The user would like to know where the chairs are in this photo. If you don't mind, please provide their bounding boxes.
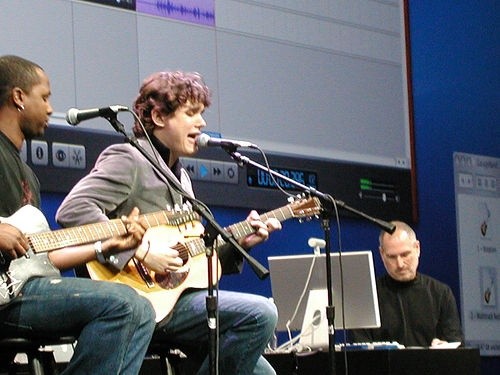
[0,261,199,375]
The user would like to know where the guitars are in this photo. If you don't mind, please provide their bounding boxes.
[0,204,203,305]
[73,191,324,325]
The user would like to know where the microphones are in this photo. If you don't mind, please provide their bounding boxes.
[196,133,258,148]
[66,105,128,126]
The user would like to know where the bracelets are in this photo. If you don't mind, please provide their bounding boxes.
[138,239,150,262]
[95,240,106,264]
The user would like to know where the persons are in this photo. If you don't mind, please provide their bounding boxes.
[0,56,157,375]
[348,221,464,349]
[55,72,281,375]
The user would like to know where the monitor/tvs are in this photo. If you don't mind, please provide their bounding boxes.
[268,251,381,353]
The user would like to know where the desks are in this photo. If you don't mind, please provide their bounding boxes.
[262,348,482,375]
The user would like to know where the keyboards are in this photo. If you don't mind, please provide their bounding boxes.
[334,340,405,351]
